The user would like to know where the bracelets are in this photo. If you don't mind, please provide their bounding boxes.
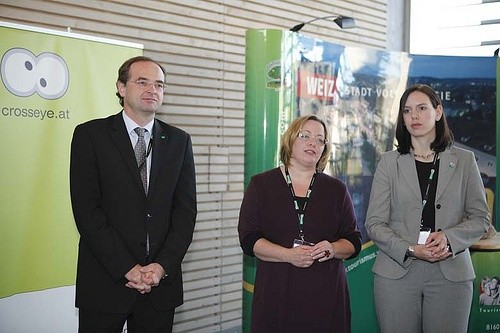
[162,274,168,281]
[407,244,414,259]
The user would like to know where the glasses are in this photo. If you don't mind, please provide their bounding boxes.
[296,131,329,147]
[125,79,168,92]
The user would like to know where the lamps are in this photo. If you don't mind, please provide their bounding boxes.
[289,15,355,32]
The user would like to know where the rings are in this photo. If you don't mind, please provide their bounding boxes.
[323,250,330,258]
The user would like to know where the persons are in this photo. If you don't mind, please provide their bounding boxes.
[69,56,198,333]
[478,277,500,305]
[364,84,491,333]
[237,115,362,333]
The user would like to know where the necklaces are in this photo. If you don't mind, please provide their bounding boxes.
[413,152,434,159]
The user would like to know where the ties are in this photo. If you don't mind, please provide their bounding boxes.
[132,128,148,197]
